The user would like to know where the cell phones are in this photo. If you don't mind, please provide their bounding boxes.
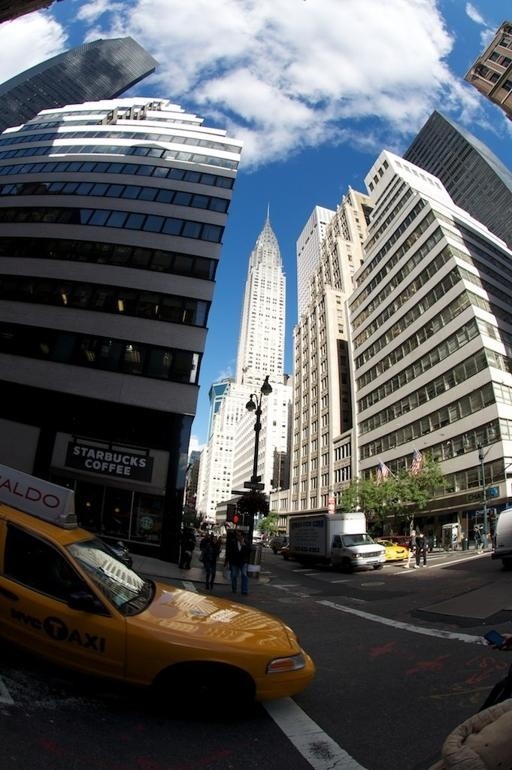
[484,630,504,651]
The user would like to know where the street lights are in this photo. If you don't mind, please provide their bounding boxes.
[245,374,273,492]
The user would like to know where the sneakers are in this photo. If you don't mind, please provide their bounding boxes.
[403,564,427,568]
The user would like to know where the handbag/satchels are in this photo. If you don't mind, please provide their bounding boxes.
[199,552,203,561]
[223,566,232,581]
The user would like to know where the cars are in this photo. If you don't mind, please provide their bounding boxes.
[252,530,290,554]
[373,534,434,562]
[0,462,316,701]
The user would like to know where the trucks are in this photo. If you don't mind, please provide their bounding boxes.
[289,512,385,571]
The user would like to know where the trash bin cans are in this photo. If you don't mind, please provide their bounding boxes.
[247,544,262,579]
[462,538,469,550]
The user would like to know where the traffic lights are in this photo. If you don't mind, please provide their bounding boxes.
[227,503,252,526]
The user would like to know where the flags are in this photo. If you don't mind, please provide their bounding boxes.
[380,461,388,482]
[376,468,381,486]
[410,450,422,475]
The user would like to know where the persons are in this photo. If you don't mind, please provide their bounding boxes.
[452,525,459,551]
[479,633,512,711]
[179,526,196,568]
[403,530,427,569]
[260,532,266,547]
[473,527,480,549]
[227,530,252,595]
[198,532,222,590]
